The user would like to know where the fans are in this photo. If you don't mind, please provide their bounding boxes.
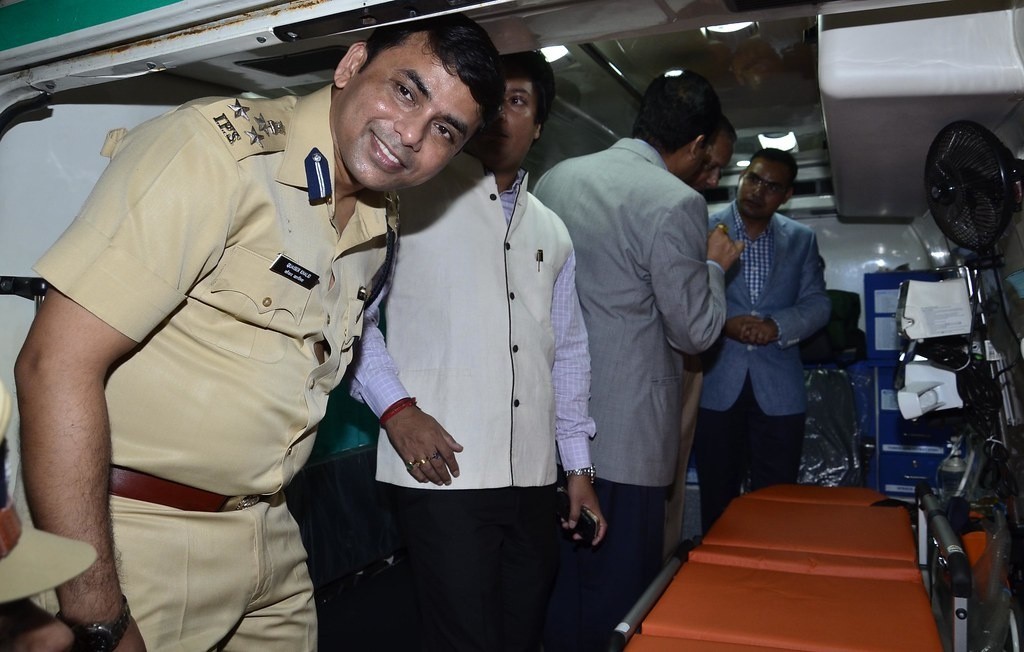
[926,117,1024,256]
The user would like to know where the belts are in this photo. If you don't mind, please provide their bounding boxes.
[108,465,260,513]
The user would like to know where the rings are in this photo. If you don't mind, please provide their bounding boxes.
[716,223,729,234]
[417,458,429,468]
[405,460,418,471]
[428,450,441,461]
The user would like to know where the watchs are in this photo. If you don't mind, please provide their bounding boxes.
[53,594,131,652]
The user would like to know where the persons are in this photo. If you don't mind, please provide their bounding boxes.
[350,50,607,652]
[1,382,99,652]
[15,3,507,652]
[692,147,834,536]
[691,114,738,193]
[533,70,749,652]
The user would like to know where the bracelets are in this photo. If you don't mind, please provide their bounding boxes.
[566,462,596,484]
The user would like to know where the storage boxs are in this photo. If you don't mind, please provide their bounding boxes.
[856,267,961,502]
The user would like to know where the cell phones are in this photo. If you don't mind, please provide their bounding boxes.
[554,483,599,544]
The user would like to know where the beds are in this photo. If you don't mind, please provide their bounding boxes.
[612,481,979,652]
